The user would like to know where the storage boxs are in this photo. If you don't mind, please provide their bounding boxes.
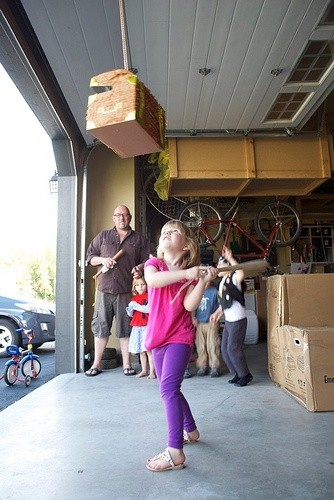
[254,273,334,413]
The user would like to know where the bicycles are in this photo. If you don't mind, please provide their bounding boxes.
[0,329,41,387]
[177,200,302,276]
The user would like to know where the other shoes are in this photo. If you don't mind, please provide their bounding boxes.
[210,367,220,377]
[184,368,191,378]
[198,368,208,375]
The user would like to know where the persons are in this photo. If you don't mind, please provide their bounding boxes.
[126,276,156,379]
[210,245,253,386]
[144,220,220,472]
[85,206,144,376]
[191,282,222,377]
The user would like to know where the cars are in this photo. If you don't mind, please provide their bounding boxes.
[0,296,55,358]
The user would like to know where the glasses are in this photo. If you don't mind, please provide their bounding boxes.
[114,213,130,218]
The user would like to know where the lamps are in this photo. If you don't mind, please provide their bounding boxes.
[48,170,58,195]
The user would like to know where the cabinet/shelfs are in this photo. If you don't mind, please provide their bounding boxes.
[241,220,334,274]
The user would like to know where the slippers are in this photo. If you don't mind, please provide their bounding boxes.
[85,369,102,376]
[181,432,199,444]
[146,449,185,472]
[123,368,135,375]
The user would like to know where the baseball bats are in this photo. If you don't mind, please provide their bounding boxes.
[92,248,125,279]
[218,258,268,273]
